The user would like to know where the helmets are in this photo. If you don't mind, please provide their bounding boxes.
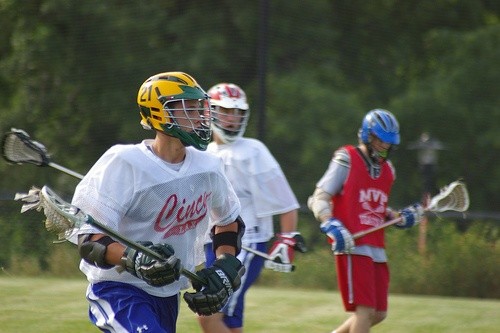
[359,108,402,148]
[135,71,212,151]
[205,82,251,144]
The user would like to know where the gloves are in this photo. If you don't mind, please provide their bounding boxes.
[318,218,357,255]
[183,253,247,316]
[119,240,183,288]
[389,203,424,229]
[263,231,308,274]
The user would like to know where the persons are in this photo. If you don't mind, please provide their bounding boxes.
[308,108,428,333]
[183,82,299,333]
[67,71,241,333]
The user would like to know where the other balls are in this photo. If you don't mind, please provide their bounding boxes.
[45,216,63,234]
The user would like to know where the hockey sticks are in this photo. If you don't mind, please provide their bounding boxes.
[14,186,209,287]
[2,128,277,260]
[328,181,471,244]
